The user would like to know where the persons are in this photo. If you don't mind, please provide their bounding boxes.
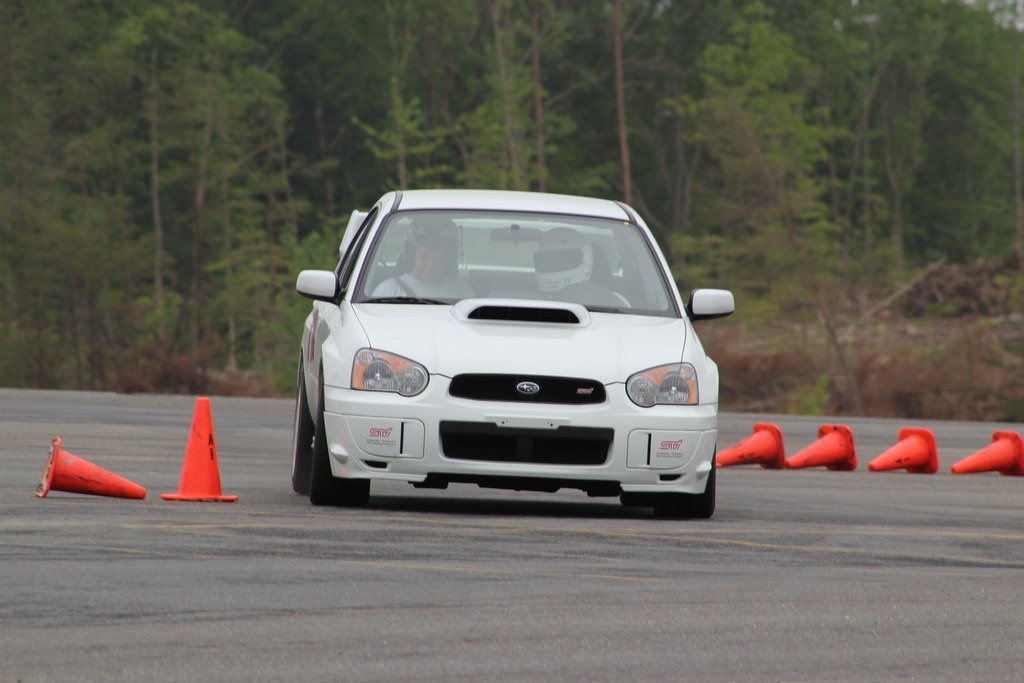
[372,214,476,299]
[523,226,604,305]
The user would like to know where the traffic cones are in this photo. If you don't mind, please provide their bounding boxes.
[35,437,147,499]
[868,427,938,473]
[786,424,857,471]
[161,398,238,500]
[951,430,1024,476]
[715,422,786,469]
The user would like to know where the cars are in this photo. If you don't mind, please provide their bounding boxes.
[292,189,735,519]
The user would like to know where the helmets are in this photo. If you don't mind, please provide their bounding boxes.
[533,227,594,292]
[404,215,461,249]
